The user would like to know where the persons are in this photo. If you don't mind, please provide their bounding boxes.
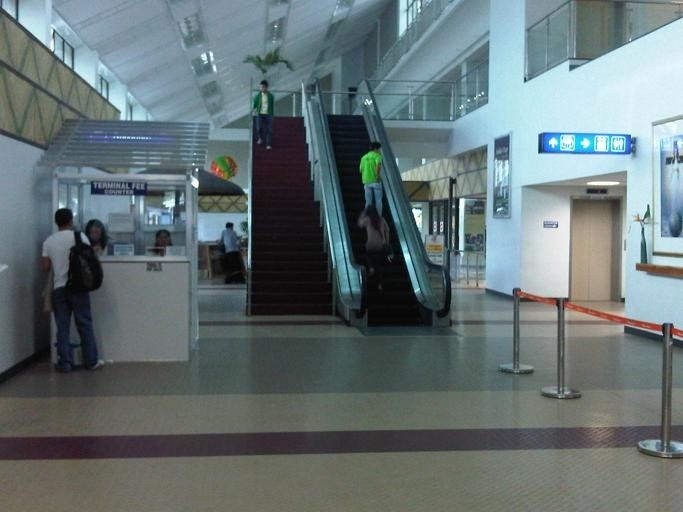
[358,205,390,290]
[251,80,274,151]
[41,209,105,373]
[218,222,241,254]
[153,230,172,256]
[85,219,112,256]
[359,142,383,216]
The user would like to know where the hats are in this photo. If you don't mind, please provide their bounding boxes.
[369,142,381,150]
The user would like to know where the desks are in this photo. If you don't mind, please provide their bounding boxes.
[197,235,247,279]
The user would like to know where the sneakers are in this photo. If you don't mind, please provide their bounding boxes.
[257,138,272,150]
[91,360,104,369]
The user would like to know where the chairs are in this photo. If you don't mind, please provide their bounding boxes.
[220,236,245,284]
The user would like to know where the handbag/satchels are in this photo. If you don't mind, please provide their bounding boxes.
[380,251,395,264]
[66,230,103,294]
[217,243,225,254]
